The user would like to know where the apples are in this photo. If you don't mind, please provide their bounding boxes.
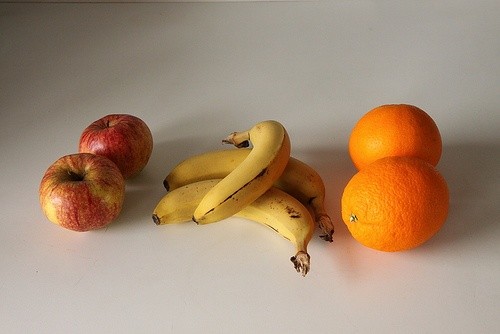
[78,114,153,178]
[38,153,124,232]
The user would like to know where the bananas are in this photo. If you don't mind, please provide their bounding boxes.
[152,179,316,277]
[163,148,334,242]
[193,119,291,225]
